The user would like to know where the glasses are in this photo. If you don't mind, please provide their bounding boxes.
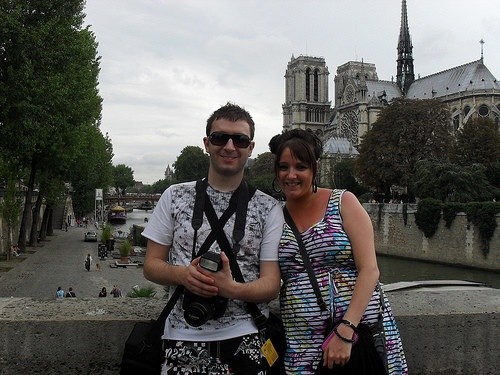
[209,131,252,150]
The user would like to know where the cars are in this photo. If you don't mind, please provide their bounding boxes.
[83,231,98,242]
[105,206,127,225]
[122,202,154,212]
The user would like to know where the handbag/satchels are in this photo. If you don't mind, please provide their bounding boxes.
[120,319,163,375]
[314,318,388,375]
[264,309,287,374]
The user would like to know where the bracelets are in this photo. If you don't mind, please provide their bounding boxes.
[335,329,354,343]
[338,320,359,333]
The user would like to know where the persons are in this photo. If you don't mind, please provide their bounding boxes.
[63,220,68,232]
[144,217,148,223]
[76,214,99,229]
[84,253,93,271]
[55,286,65,298]
[110,284,122,298]
[141,104,287,375]
[99,287,107,298]
[269,127,411,375]
[66,287,76,297]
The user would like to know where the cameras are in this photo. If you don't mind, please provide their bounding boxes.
[182,252,228,327]
